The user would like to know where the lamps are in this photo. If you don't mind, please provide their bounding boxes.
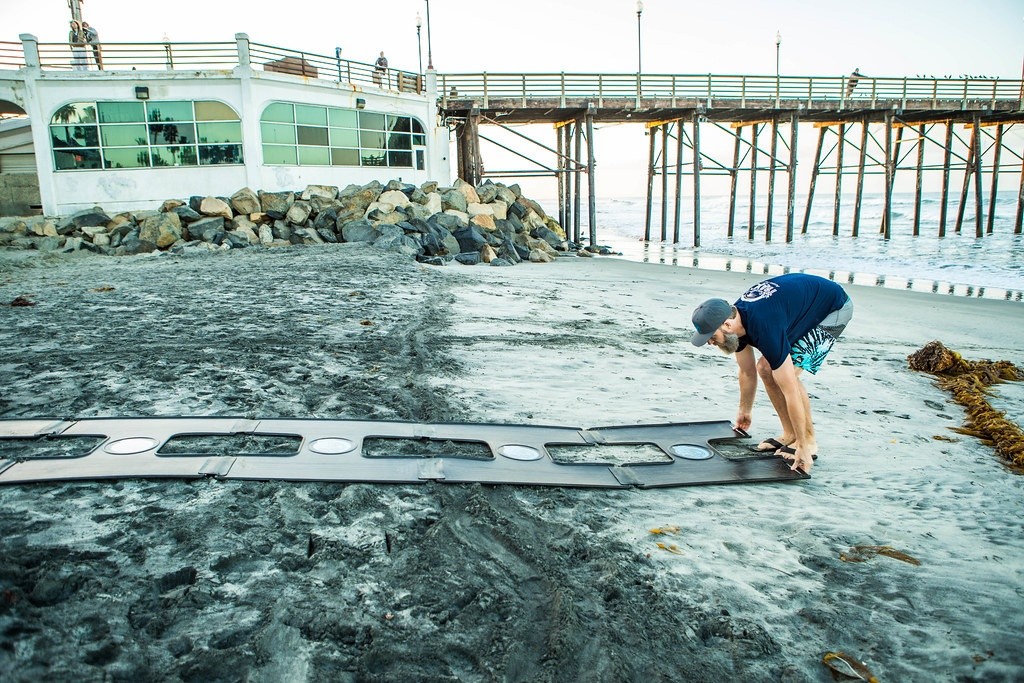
[357,99,366,109]
[135,87,149,99]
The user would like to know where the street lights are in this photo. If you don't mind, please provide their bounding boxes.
[774,29,784,110]
[636,0,645,98]
[414,12,424,91]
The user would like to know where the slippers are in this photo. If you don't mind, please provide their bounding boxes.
[748,438,818,463]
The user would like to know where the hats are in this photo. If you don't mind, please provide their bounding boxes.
[691,298,731,347]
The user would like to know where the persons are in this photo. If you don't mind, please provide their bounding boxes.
[374,51,389,87]
[68,20,105,73]
[689,271,854,476]
[845,68,869,98]
